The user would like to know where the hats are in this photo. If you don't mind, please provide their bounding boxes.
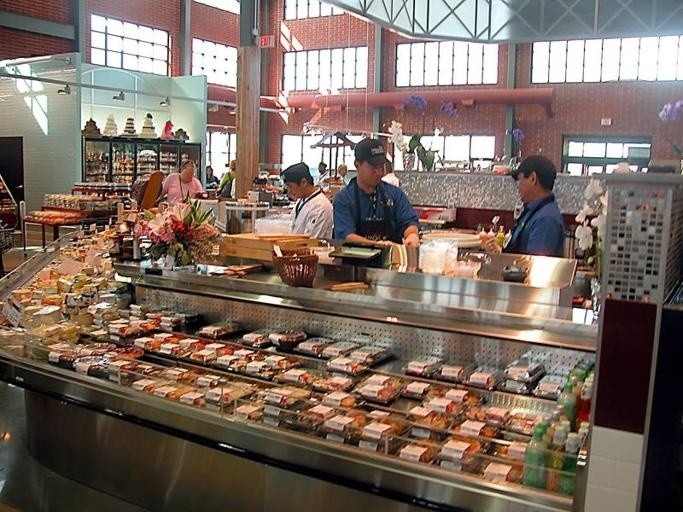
[354,138,389,166]
[281,162,309,181]
[509,155,556,178]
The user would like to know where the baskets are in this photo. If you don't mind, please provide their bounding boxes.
[272,249,318,286]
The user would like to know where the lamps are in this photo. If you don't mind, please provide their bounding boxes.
[58,84,72,95]
[160,97,172,107]
[113,92,125,101]
[208,104,219,111]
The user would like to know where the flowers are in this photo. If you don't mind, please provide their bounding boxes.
[387,120,422,152]
[133,191,224,273]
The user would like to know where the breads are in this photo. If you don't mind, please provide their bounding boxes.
[1,276,561,483]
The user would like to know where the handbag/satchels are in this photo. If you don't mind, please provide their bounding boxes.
[221,172,232,197]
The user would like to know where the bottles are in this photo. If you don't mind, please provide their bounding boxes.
[476,222,512,251]
[523,358,596,495]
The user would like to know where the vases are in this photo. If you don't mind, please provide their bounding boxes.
[403,152,415,172]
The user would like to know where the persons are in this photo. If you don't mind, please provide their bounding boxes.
[217,161,237,197]
[157,162,203,213]
[283,162,334,240]
[205,166,218,187]
[316,163,329,183]
[495,154,566,258]
[336,164,349,188]
[382,160,399,188]
[332,138,420,250]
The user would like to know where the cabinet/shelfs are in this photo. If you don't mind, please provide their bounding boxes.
[22,187,120,250]
[80,135,201,192]
[1,230,600,511]
